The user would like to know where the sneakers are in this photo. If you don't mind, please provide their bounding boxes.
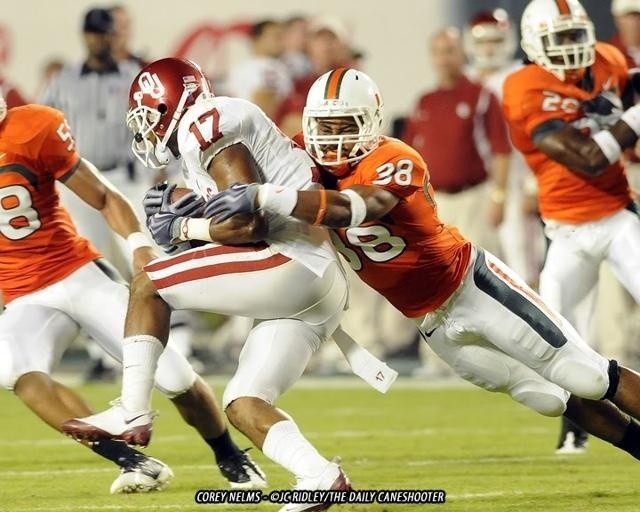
[216,454,267,495]
[57,413,156,447]
[555,418,591,460]
[108,458,172,494]
[276,463,355,508]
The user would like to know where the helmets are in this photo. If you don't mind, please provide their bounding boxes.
[462,10,516,72]
[302,68,383,161]
[124,56,215,172]
[517,0,597,79]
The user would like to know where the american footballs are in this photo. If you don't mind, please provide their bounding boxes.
[170,188,211,246]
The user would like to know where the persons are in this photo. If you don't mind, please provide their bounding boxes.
[2,0,640,511]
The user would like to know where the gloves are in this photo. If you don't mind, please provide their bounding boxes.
[145,182,254,248]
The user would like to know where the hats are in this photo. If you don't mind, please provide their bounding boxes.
[84,8,115,35]
